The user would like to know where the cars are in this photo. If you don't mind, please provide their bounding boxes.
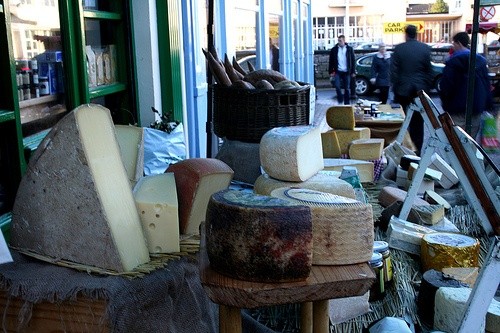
[329,50,447,97]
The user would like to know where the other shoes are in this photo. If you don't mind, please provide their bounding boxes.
[338,94,343,104]
[344,97,351,105]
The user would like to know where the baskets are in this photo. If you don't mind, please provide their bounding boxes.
[210,80,309,143]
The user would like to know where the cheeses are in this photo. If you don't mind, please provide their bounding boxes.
[10,102,233,275]
[205,106,480,333]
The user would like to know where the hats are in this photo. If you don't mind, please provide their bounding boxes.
[379,42,386,47]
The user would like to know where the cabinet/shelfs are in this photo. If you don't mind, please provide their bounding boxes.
[0,0,135,244]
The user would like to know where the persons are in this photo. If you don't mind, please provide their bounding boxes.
[439,32,490,142]
[327,34,356,105]
[388,25,434,153]
[371,42,394,104]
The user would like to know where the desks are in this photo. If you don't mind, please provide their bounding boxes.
[354,104,405,138]
[199,222,376,333]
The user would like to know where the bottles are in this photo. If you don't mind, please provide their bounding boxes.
[373,240,393,288]
[367,252,384,303]
[14,58,49,102]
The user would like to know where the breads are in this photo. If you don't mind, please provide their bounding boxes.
[202,47,300,90]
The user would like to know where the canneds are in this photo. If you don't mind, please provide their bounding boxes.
[16,58,49,100]
